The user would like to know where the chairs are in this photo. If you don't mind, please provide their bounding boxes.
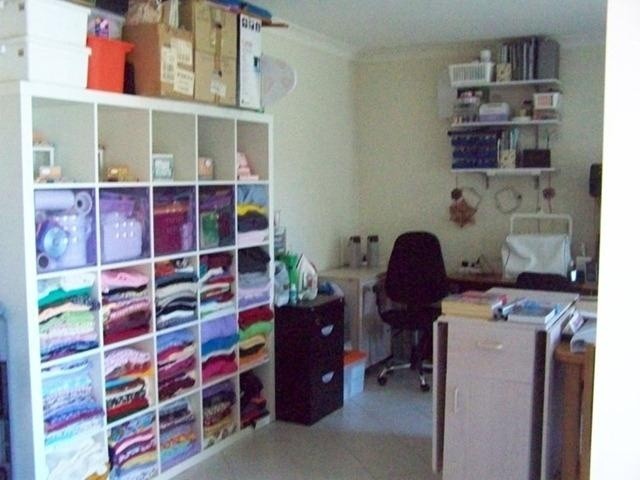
[372,229,449,393]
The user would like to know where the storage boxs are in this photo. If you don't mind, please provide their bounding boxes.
[344,352,367,399]
[0,0,262,115]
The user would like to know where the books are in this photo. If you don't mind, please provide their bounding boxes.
[500,40,559,79]
[508,306,556,324]
[440,291,506,320]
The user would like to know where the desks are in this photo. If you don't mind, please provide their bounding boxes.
[556,336,591,480]
[450,272,505,295]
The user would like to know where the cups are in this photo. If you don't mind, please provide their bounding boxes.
[500,148,516,169]
[496,63,513,82]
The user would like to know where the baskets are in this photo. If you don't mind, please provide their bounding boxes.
[101,217,142,262]
[92,191,136,218]
[52,213,87,269]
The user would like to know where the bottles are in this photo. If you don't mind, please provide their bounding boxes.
[365,234,381,269]
[520,109,528,116]
[567,260,577,288]
[460,261,469,276]
[347,234,362,270]
[290,283,298,304]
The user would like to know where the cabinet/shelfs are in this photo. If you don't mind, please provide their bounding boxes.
[318,260,391,373]
[431,289,578,480]
[276,296,345,427]
[0,81,278,479]
[450,80,563,175]
